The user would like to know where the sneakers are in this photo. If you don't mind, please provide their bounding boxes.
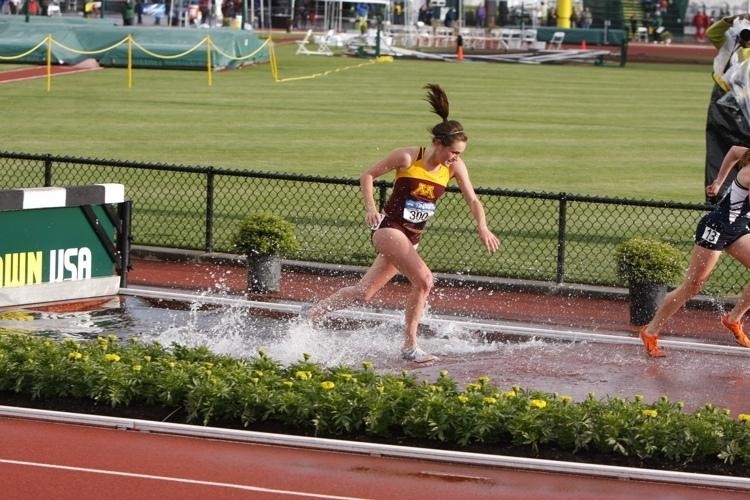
[721,314,750,348]
[300,304,313,321]
[639,325,667,358]
[400,346,437,363]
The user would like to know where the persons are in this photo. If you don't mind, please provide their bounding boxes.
[9,0,234,27]
[353,0,593,34]
[456,35,464,61]
[299,120,500,366]
[630,0,750,42]
[704,13,750,204]
[639,145,750,357]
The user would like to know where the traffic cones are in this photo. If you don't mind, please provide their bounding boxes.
[580,39,587,49]
[457,46,465,62]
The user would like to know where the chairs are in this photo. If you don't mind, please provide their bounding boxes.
[294,25,569,57]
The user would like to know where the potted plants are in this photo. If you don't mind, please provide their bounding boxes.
[613,238,690,330]
[230,210,299,292]
